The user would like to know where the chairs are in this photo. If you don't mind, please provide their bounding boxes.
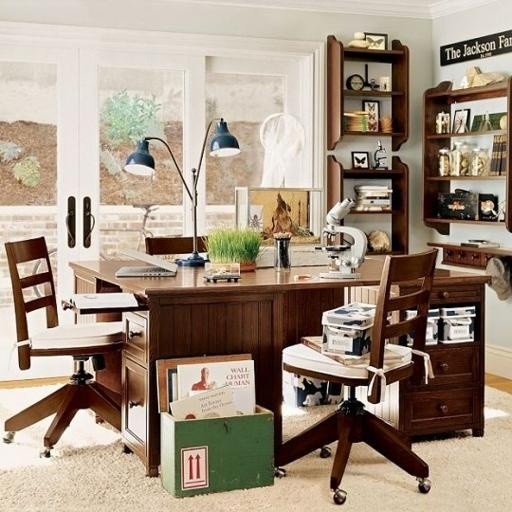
[269,242,438,504]
[143,234,209,262]
[5,235,127,455]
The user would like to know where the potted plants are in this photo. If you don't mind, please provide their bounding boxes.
[202,224,262,274]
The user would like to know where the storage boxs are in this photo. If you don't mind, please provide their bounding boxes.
[319,299,393,357]
[286,367,344,408]
[435,189,498,222]
[443,302,478,344]
[401,308,441,347]
[153,404,276,500]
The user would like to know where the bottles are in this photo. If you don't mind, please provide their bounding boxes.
[436,141,489,177]
[273,231,292,273]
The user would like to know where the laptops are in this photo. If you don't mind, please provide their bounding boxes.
[115,249,178,277]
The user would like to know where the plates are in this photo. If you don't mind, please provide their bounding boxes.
[369,230,389,250]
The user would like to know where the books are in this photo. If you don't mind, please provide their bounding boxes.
[71,291,139,310]
[167,359,257,418]
[300,334,404,369]
[489,134,509,177]
[353,186,392,212]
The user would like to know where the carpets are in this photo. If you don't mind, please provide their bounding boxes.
[1,375,511,510]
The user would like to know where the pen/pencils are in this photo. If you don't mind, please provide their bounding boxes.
[273,231,293,269]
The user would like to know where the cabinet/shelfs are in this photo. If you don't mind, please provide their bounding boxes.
[422,72,512,236]
[326,155,412,256]
[325,30,412,151]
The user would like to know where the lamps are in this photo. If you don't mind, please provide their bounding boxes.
[121,113,240,268]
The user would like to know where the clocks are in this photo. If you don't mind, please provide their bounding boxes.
[346,73,365,91]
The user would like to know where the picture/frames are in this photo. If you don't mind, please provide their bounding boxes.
[352,151,371,170]
[361,99,380,133]
[364,32,389,51]
[453,108,471,134]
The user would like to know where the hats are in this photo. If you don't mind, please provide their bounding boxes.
[486,257,510,300]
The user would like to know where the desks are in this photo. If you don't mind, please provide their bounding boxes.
[66,246,496,441]
